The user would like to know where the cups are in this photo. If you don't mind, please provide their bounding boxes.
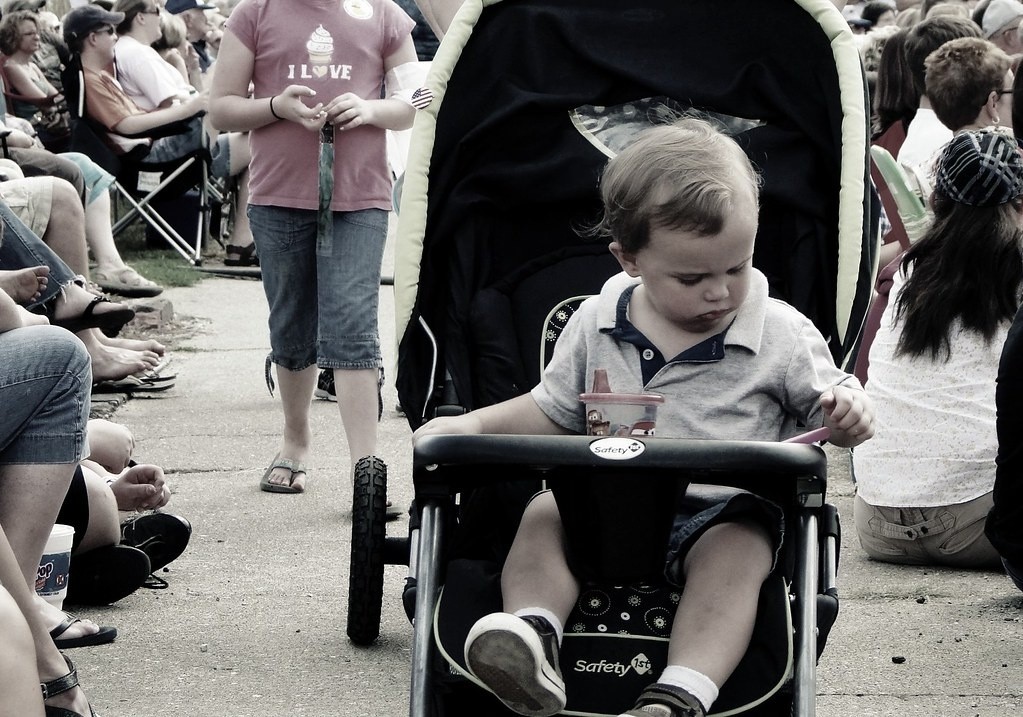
[577,370,664,437]
[36,523,75,611]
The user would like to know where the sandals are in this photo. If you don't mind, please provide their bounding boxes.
[49,610,117,649]
[40,650,98,717]
[94,266,164,298]
[224,241,260,267]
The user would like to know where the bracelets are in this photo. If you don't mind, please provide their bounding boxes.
[270,96,285,121]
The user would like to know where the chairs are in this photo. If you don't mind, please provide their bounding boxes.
[0,54,231,267]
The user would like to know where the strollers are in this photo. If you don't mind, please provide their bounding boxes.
[346,1,898,717]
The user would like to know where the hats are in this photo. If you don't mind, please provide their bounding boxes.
[165,0,215,14]
[981,0,1023,41]
[934,130,1023,208]
[63,4,125,43]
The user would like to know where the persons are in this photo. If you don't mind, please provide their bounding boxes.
[209,0,423,515]
[0,0,260,717]
[833,0,1023,594]
[409,120,877,717]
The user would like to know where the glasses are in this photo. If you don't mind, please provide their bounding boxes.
[94,26,113,35]
[21,29,41,36]
[141,7,159,16]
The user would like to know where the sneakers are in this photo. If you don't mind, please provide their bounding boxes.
[464,612,567,717]
[617,683,706,717]
[315,372,337,401]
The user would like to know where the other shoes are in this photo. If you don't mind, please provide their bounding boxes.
[65,513,191,611]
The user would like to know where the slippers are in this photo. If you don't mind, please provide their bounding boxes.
[51,296,135,334]
[260,451,306,493]
[91,377,175,394]
[140,369,176,383]
